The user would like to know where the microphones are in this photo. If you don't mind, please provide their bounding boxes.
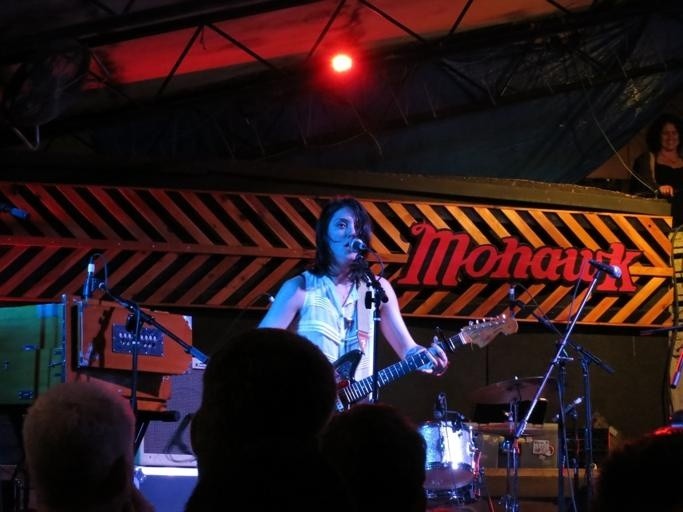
[83,263,97,302]
[348,238,366,256]
[508,288,516,319]
[590,258,621,279]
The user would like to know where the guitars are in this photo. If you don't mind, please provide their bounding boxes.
[335,310,520,422]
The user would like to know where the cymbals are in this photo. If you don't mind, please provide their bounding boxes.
[478,426,545,438]
[470,376,555,404]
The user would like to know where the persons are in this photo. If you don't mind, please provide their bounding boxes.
[312,402,429,511]
[587,425,683,512]
[630,113,683,231]
[256,197,450,416]
[183,326,337,511]
[22,379,158,512]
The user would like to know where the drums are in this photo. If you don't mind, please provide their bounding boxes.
[416,418,476,492]
[426,485,480,503]
